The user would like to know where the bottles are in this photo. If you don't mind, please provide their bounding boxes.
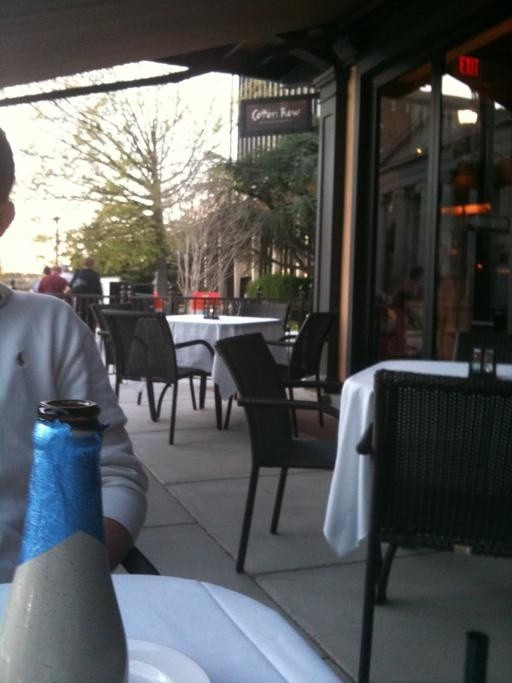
[0,398,130,683]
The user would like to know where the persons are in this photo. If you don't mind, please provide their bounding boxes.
[390,265,424,308]
[32,258,104,338]
[0,126,149,584]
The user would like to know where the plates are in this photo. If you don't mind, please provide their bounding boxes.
[124,639,211,683]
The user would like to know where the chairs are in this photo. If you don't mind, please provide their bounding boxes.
[36,280,159,357]
[224,310,342,429]
[99,308,223,443]
[213,332,334,574]
[194,301,292,411]
[356,369,511,682]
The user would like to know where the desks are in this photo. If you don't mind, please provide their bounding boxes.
[322,358,510,562]
[159,311,285,427]
[0,571,355,683]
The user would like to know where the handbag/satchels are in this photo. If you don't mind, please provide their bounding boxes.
[71,268,87,292]
